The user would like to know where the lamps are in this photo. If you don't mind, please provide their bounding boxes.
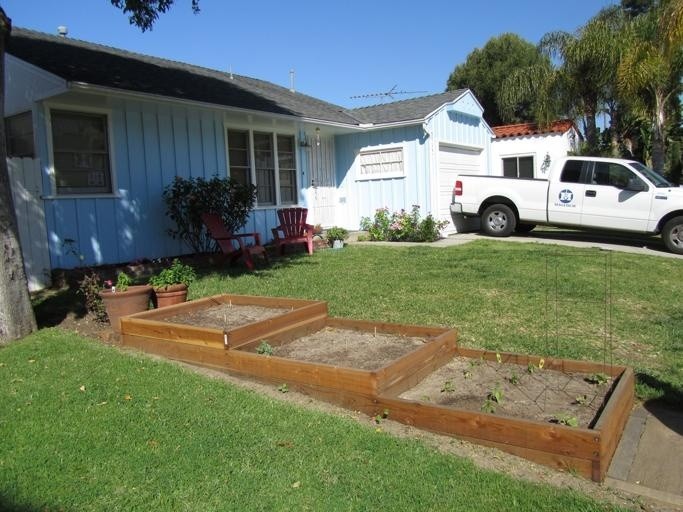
[315,125,320,147]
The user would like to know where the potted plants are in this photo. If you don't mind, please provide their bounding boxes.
[97,257,196,336]
[160,176,258,271]
[326,225,350,249]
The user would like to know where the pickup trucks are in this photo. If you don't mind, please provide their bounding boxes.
[451,155,682,254]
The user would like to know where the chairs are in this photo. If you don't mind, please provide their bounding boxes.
[202,206,314,271]
